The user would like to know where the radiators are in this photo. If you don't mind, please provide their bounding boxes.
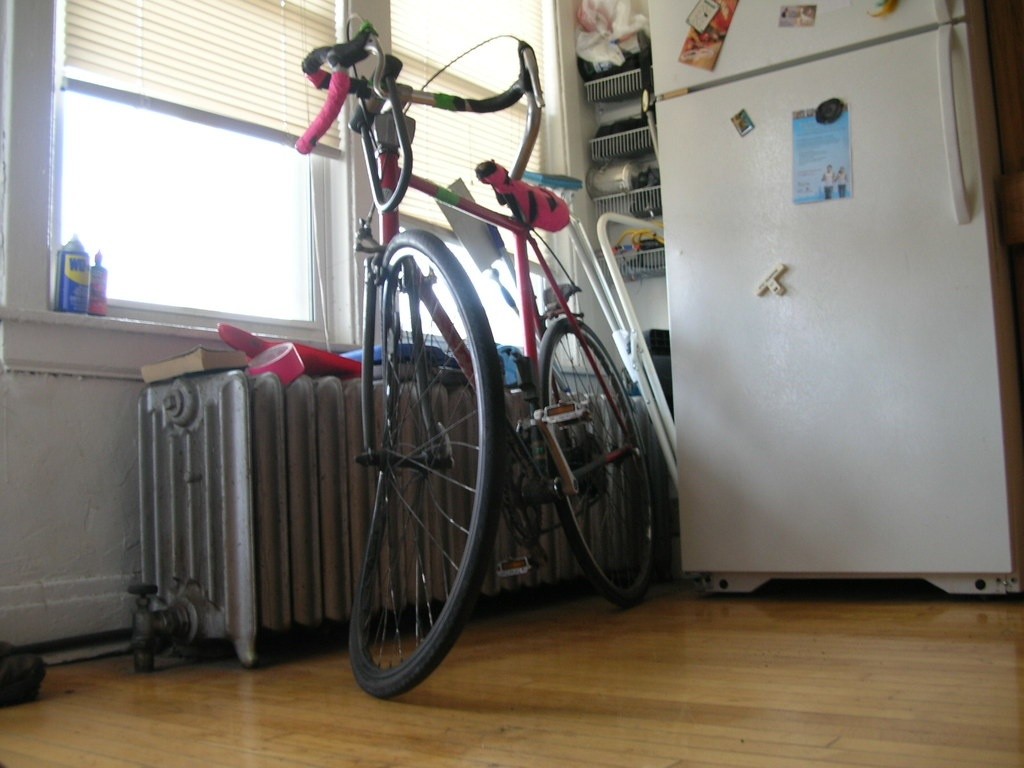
[126,365,674,676]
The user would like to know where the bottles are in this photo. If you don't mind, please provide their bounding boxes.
[54,234,88,315]
[86,251,108,317]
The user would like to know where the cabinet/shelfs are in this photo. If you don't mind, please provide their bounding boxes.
[577,29,665,282]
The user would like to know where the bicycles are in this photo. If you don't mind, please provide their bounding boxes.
[292,13,672,703]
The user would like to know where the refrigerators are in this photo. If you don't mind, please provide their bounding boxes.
[650,1,1023,599]
